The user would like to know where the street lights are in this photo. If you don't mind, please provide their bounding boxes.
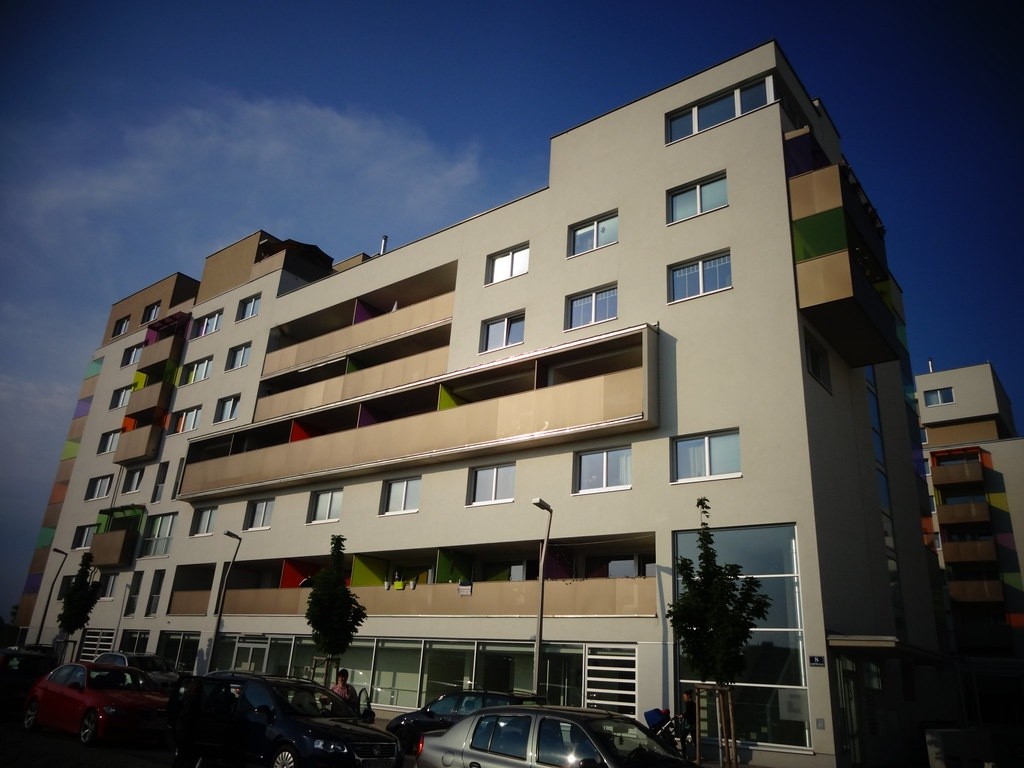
[531,497,552,698]
[204,529,242,677]
[32,546,68,642]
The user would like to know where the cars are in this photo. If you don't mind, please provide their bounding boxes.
[414,704,705,768]
[0,647,52,722]
[381,692,564,756]
[24,661,171,747]
[169,670,403,768]
[90,652,181,680]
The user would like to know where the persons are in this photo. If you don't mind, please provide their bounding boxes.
[319,669,357,717]
[676,691,696,760]
